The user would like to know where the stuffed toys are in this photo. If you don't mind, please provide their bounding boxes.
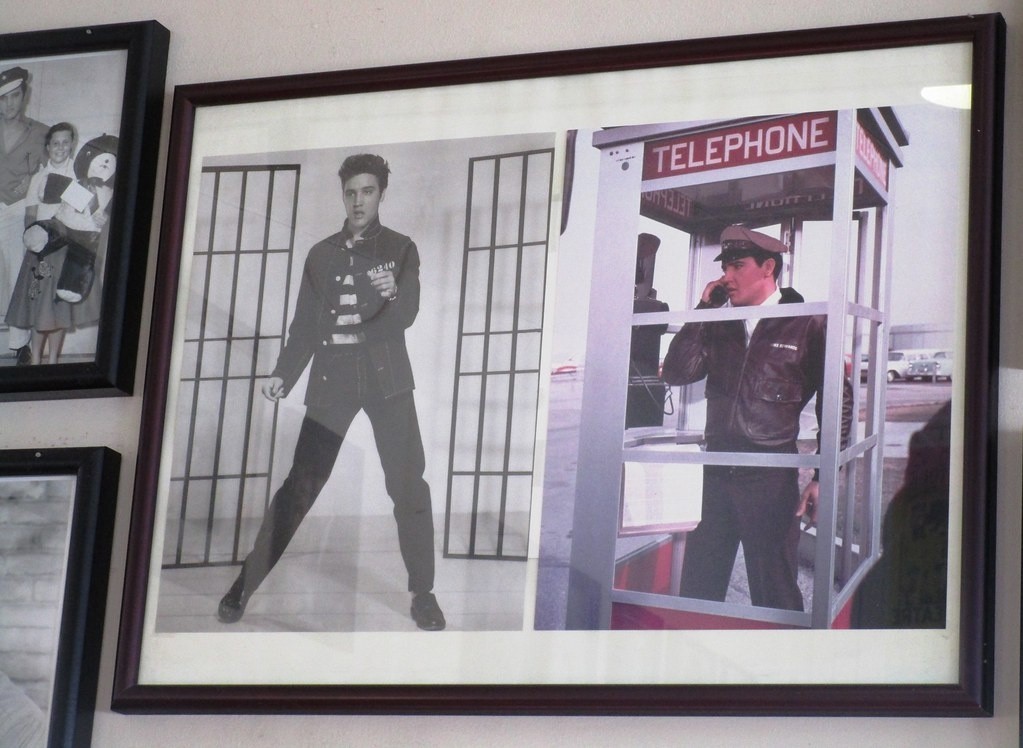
[23,135,119,303]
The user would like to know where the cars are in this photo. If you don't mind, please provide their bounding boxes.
[844,346,955,381]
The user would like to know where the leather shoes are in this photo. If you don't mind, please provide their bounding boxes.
[218,578,252,624]
[411,592,446,632]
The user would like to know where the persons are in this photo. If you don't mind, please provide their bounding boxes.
[662,226,853,614]
[218,155,447,631]
[0,66,97,367]
[851,399,951,631]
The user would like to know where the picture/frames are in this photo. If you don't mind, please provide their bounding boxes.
[0,446,122,748]
[0,20,172,403]
[111,11,1008,717]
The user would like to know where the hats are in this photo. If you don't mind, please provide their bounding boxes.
[712,226,788,262]
[0,67,28,98]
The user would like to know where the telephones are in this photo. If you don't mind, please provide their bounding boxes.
[709,284,730,307]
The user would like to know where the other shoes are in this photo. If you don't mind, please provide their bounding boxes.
[13,345,31,367]
